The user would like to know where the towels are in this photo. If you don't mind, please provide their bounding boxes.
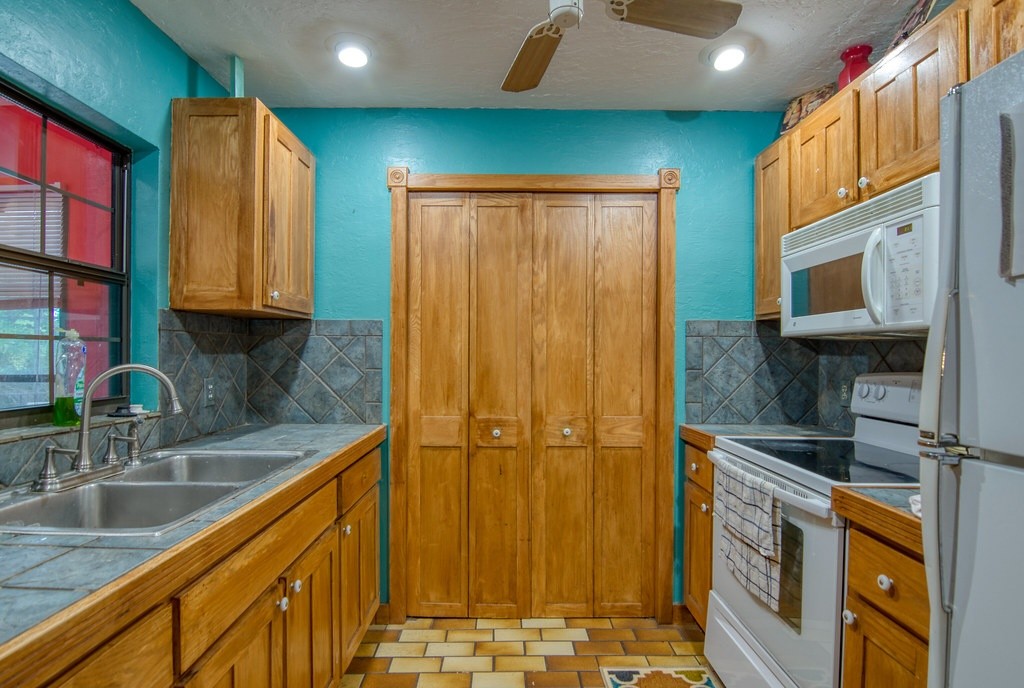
[712,456,782,614]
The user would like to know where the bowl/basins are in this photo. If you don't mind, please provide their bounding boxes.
[908,495,921,519]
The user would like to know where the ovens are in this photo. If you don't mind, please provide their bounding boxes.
[704,446,846,688]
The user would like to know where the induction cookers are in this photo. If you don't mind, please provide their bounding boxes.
[714,372,923,499]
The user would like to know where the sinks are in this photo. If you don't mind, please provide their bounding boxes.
[0,483,244,529]
[101,454,298,482]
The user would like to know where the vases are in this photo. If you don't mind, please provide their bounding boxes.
[839,44,872,92]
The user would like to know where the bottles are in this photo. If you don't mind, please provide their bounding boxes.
[53,326,86,428]
[838,44,872,92]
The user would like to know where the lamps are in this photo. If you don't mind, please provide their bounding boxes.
[336,41,370,67]
[710,45,745,72]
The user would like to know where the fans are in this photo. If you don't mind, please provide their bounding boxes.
[501,0,743,93]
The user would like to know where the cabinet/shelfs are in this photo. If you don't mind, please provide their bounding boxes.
[754,137,790,320]
[337,447,382,676]
[968,0,1024,79]
[843,527,930,688]
[170,97,315,319]
[45,602,174,688]
[172,477,341,688]
[684,445,714,633]
[790,9,967,229]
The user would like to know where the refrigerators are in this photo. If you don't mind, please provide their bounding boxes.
[917,47,1024,688]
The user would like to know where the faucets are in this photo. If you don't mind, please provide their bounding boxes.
[70,364,185,470]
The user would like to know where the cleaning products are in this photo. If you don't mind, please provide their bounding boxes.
[52,326,87,427]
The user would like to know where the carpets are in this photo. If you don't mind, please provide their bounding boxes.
[599,665,719,688]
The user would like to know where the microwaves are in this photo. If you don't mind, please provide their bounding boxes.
[780,172,940,341]
